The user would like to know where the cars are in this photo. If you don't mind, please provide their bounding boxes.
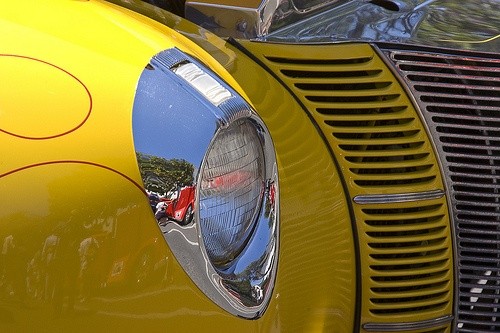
[165,186,196,226]
[0,0,500,333]
